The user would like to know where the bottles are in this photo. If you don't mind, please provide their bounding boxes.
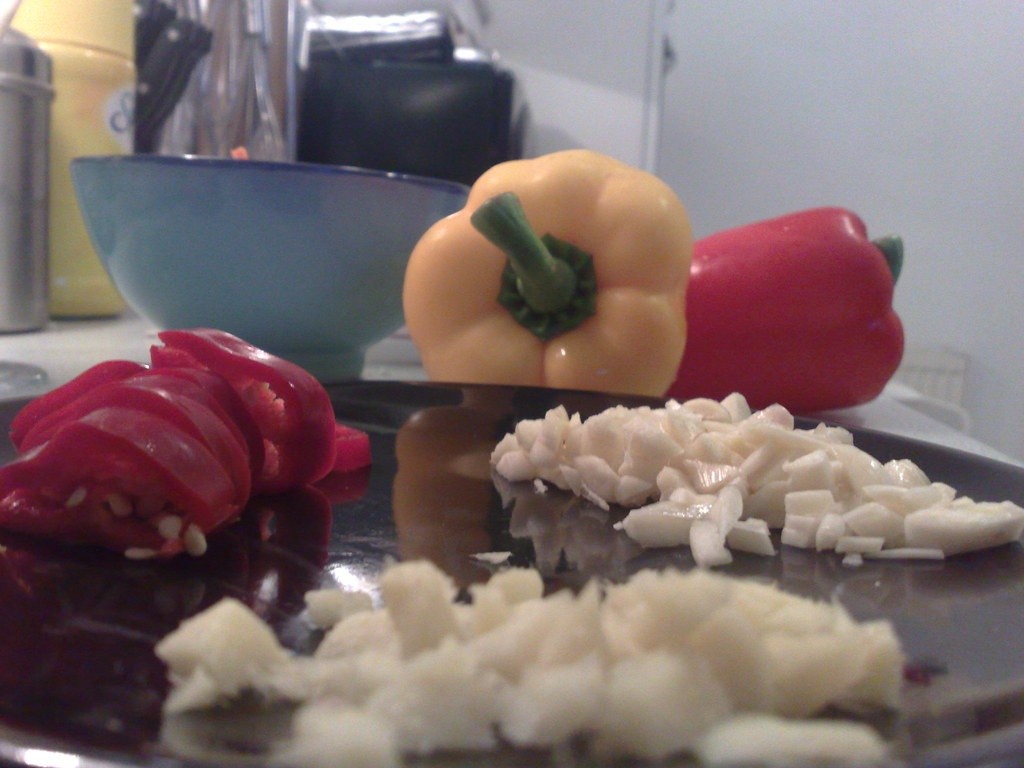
[8,0,148,317]
[0,25,55,334]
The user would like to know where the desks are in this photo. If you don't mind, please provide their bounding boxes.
[0,315,1024,768]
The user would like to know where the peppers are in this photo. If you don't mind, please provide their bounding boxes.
[0,328,370,557]
[402,150,693,396]
[668,208,906,412]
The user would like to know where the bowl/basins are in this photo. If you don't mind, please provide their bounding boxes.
[69,154,471,382]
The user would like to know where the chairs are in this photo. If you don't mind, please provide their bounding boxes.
[893,342,970,406]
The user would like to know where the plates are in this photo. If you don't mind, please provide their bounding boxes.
[0,380,1024,768]
